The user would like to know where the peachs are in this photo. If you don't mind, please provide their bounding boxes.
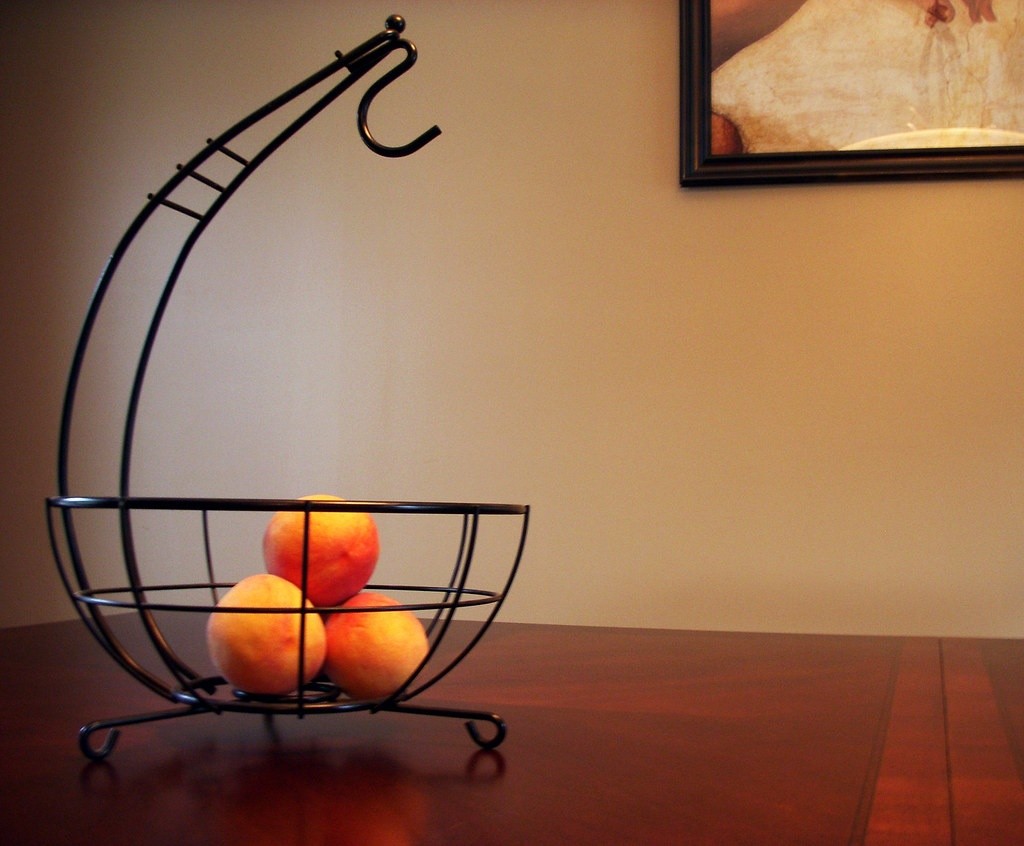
[206,494,436,703]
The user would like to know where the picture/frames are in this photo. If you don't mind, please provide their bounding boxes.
[678,0,1024,187]
